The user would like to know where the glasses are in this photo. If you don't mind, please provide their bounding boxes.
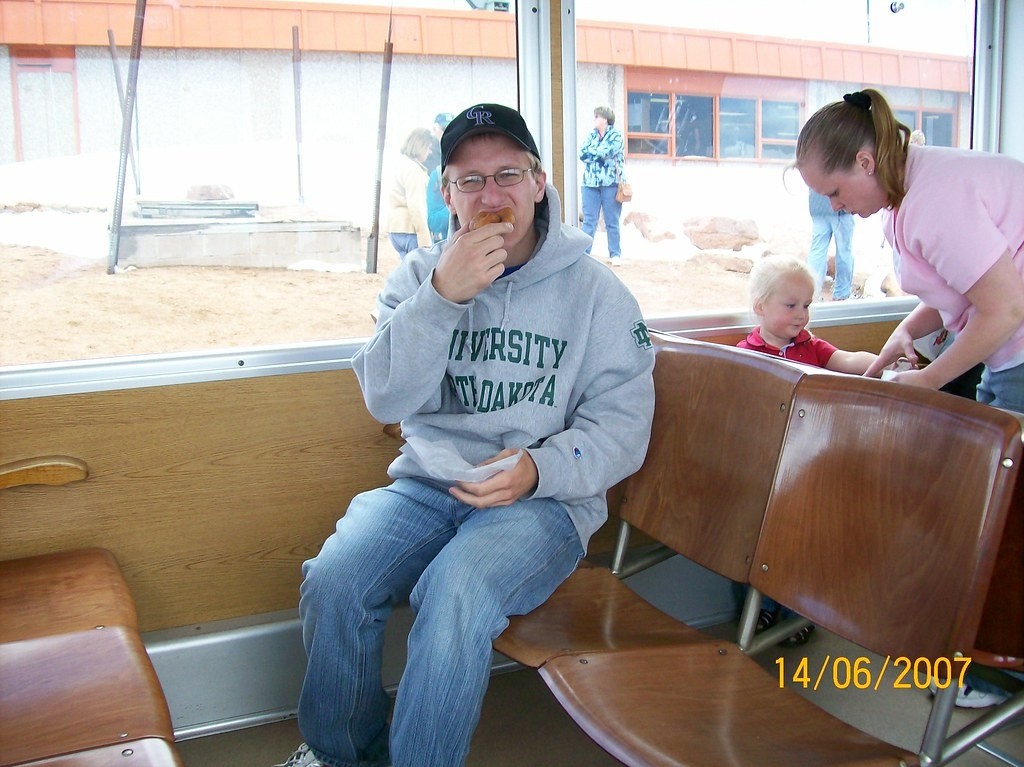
[448,168,532,193]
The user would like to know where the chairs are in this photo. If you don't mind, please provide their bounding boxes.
[0,452,186,767]
[492,330,1023,767]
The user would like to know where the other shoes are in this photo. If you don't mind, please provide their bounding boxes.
[926,676,1008,708]
[277,743,321,767]
[610,256,621,266]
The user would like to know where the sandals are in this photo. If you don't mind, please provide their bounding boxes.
[779,625,814,647]
[735,605,774,633]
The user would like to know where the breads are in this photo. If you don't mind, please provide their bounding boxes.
[468,208,515,231]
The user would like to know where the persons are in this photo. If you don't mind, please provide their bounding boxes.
[796,88,1024,708]
[274,103,654,767]
[386,128,432,261]
[427,166,450,244]
[909,129,925,146]
[578,106,625,265]
[807,187,855,301]
[736,255,919,646]
[422,113,455,177]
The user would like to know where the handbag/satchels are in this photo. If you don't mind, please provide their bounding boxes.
[615,183,633,202]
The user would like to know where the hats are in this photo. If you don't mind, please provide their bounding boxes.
[441,103,541,174]
[435,111,457,131]
